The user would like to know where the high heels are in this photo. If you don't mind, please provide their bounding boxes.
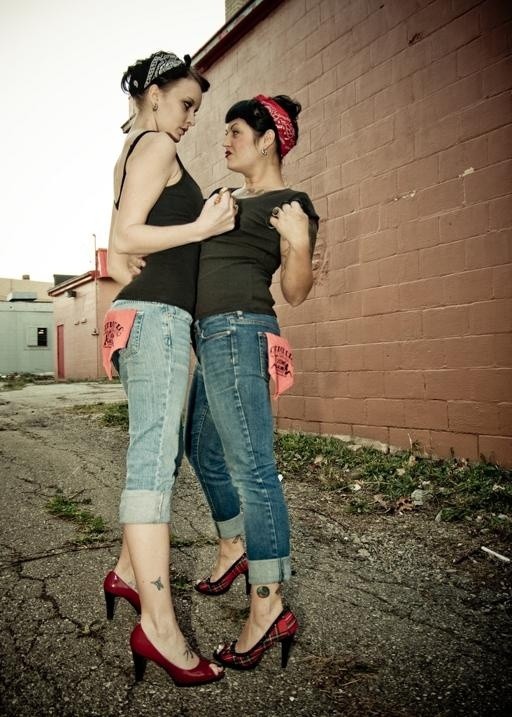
[213,607,297,670]
[104,571,141,620]
[130,624,225,687]
[194,552,251,596]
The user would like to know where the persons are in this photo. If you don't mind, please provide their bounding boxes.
[100,42,240,689]
[124,92,320,673]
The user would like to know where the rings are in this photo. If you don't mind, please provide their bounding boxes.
[272,206,280,216]
